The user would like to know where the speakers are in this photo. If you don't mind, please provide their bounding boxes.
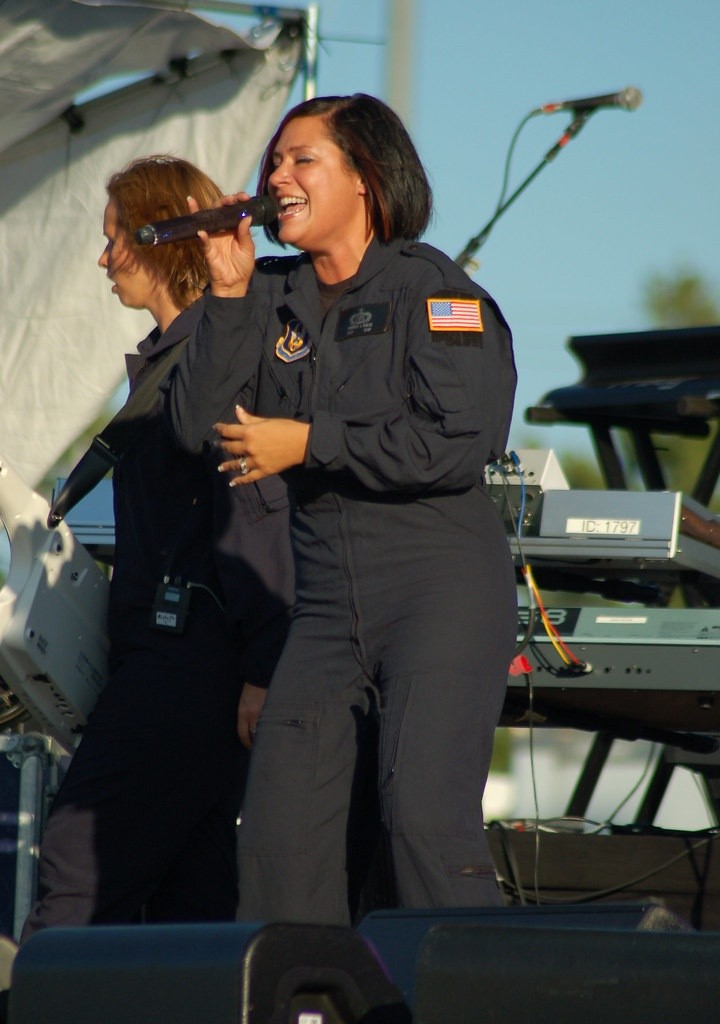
[2,900,719,1024]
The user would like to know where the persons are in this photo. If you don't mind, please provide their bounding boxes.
[186,93,522,924]
[13,154,295,988]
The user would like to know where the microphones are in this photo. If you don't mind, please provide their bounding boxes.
[533,85,643,120]
[132,195,279,246]
